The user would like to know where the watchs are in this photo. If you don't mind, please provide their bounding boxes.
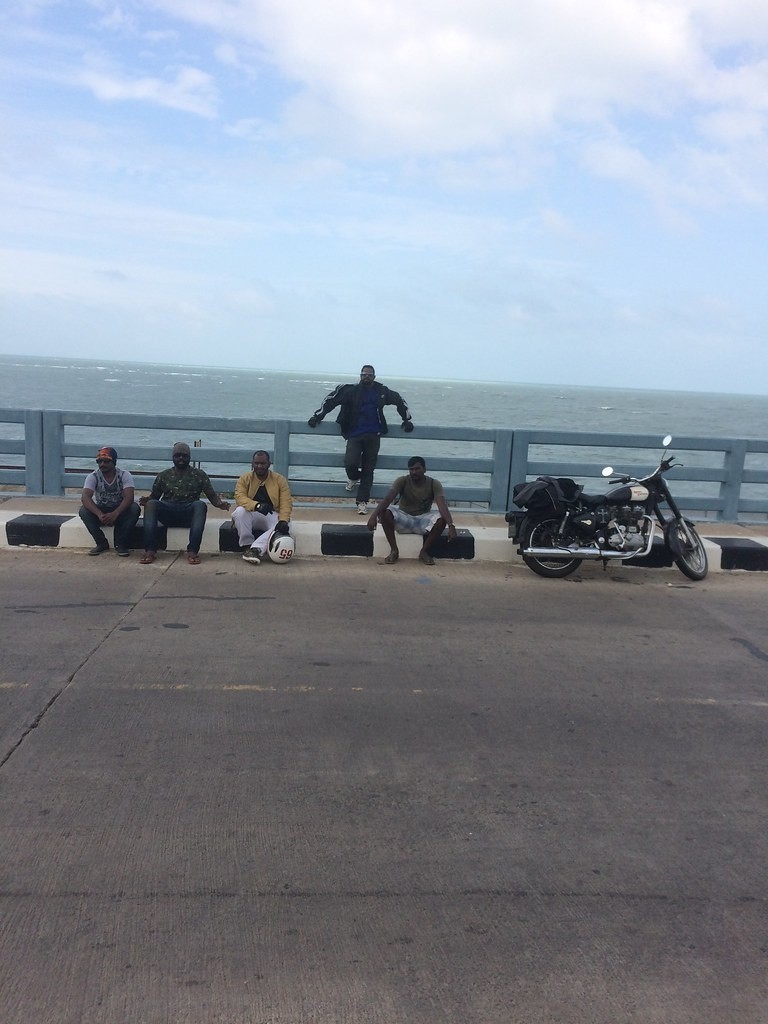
[449,523,455,527]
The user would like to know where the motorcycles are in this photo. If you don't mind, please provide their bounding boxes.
[519,435,710,579]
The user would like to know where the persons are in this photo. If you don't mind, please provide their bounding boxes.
[308,365,414,514]
[79,447,141,557]
[139,442,231,564]
[231,450,292,563]
[366,455,457,565]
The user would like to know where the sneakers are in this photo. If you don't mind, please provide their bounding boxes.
[358,502,367,515]
[345,478,359,491]
[242,548,260,565]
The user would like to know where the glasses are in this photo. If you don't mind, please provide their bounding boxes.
[97,459,111,463]
[360,373,373,377]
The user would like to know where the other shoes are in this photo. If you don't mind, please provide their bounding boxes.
[140,550,157,563]
[419,552,434,565]
[187,552,200,564]
[88,542,109,555]
[115,545,129,556]
[385,551,399,563]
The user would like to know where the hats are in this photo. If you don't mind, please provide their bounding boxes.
[172,442,190,458]
[96,447,117,465]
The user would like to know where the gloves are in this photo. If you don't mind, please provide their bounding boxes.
[308,416,321,428]
[274,521,289,534]
[401,421,413,432]
[255,503,275,516]
